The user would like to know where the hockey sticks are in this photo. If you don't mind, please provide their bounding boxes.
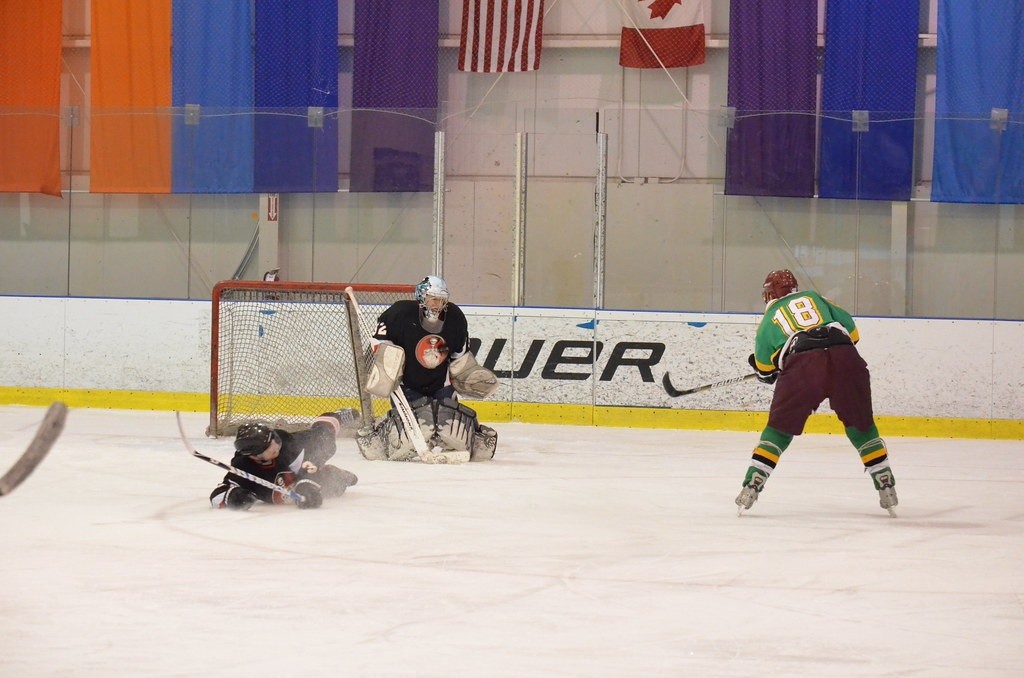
[176,410,306,504]
[0,401,68,497]
[344,284,471,465]
[662,370,757,398]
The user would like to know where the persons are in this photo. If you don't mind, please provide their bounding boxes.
[735,268,904,519]
[210,408,361,510]
[356,275,499,465]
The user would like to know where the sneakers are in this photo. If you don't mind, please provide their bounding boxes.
[326,465,358,486]
[335,409,360,427]
[876,471,899,518]
[735,470,767,517]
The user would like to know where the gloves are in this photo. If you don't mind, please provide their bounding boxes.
[224,486,257,511]
[748,353,778,384]
[295,484,323,509]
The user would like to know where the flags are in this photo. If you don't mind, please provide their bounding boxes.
[457,0,544,72]
[619,0,712,68]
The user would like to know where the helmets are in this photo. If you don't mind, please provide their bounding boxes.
[415,275,449,323]
[763,269,798,304]
[234,423,274,456]
